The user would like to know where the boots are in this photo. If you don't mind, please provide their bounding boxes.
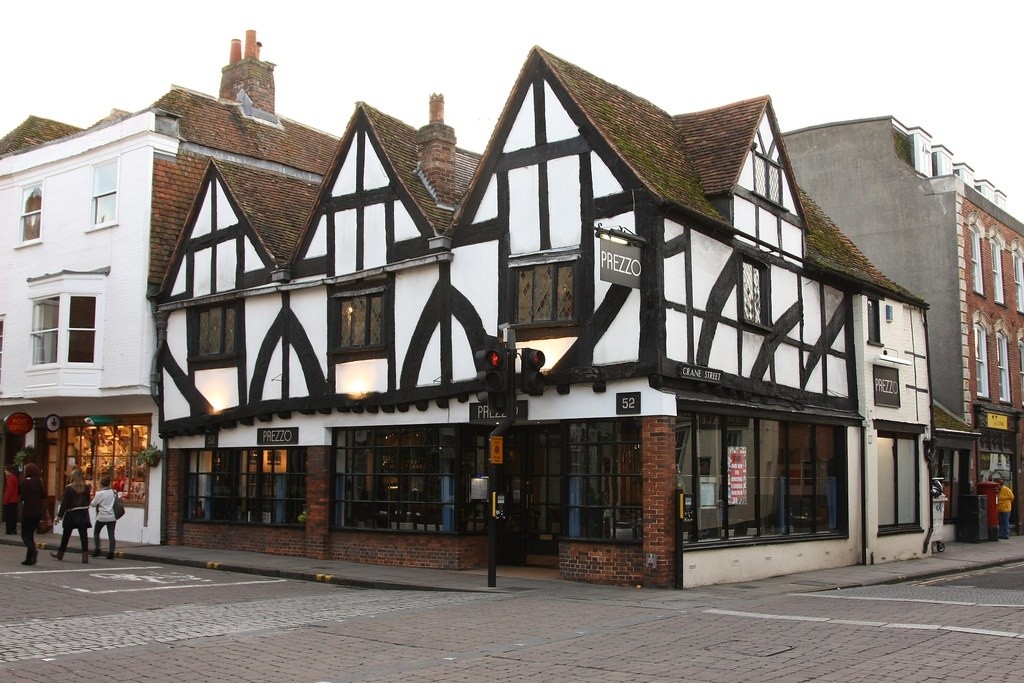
[50,550,64,560]
[81,552,88,563]
[22,550,35,565]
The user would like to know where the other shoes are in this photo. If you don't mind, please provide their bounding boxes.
[6,530,13,534]
[12,530,17,534]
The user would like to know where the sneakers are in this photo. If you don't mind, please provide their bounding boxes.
[1001,535,1009,539]
[998,534,1004,538]
[107,553,114,559]
[91,549,101,557]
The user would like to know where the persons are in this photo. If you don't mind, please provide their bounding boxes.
[4,465,20,535]
[21,463,44,565]
[50,468,92,564]
[90,474,119,559]
[996,479,1014,539]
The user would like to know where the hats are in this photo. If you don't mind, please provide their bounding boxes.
[993,478,1004,485]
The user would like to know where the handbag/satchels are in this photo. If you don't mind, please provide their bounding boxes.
[112,490,126,521]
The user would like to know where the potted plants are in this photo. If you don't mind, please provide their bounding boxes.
[12,444,36,466]
[136,444,162,468]
